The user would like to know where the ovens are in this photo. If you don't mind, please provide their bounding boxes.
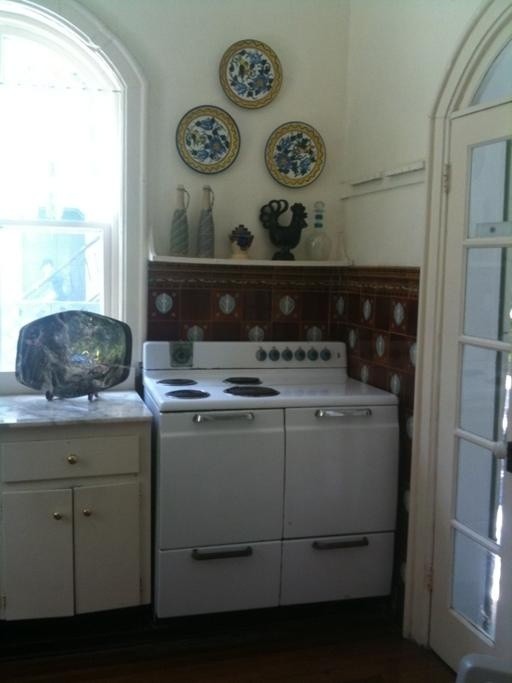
[156,408,400,619]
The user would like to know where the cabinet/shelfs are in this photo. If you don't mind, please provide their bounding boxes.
[0,424,151,623]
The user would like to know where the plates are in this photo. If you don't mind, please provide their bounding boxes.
[176,104,241,174]
[264,121,327,189]
[219,38,284,109]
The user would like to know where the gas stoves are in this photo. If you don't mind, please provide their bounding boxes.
[142,340,400,411]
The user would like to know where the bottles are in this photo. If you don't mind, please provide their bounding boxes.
[196,186,215,258]
[306,201,331,262]
[169,185,190,257]
